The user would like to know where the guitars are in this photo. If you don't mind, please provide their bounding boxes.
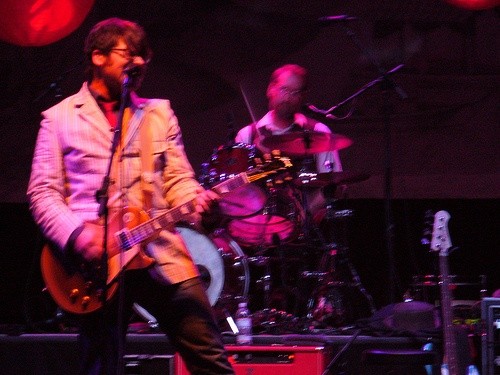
[421,209,480,375]
[41,150,293,315]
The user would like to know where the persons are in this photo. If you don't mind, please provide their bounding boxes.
[224,64,346,328]
[28,18,236,375]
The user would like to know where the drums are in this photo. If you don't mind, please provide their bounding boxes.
[225,185,305,248]
[133,223,251,328]
[206,143,270,218]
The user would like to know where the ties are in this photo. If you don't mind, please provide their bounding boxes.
[97,99,119,129]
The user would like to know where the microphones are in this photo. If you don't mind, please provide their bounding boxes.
[124,65,141,79]
[305,102,338,121]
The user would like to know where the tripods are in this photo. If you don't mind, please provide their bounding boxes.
[303,185,376,333]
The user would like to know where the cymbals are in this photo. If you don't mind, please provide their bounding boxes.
[261,131,354,154]
[294,171,370,189]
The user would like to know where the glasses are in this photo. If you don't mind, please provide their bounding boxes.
[109,48,153,63]
